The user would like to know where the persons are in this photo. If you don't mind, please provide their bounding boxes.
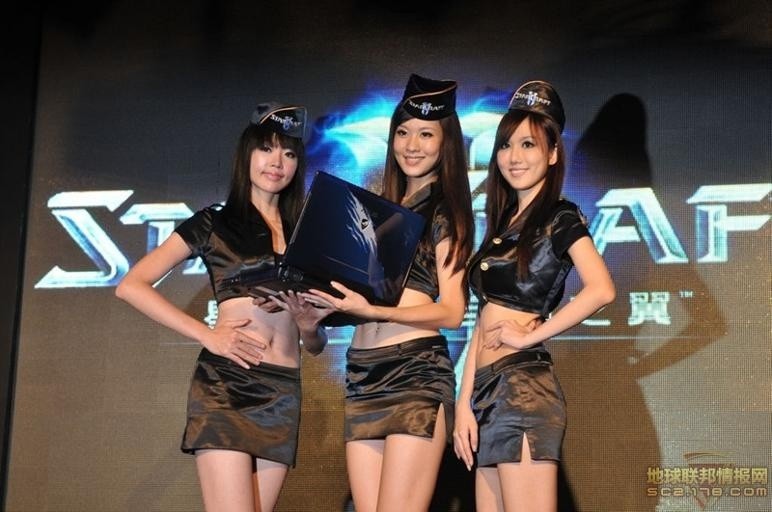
[112,101,334,512]
[252,72,474,512]
[451,79,617,512]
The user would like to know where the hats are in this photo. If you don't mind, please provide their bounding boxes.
[505,79,567,135]
[400,71,459,121]
[248,99,308,140]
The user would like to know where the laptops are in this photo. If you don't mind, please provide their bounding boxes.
[218,171,430,328]
